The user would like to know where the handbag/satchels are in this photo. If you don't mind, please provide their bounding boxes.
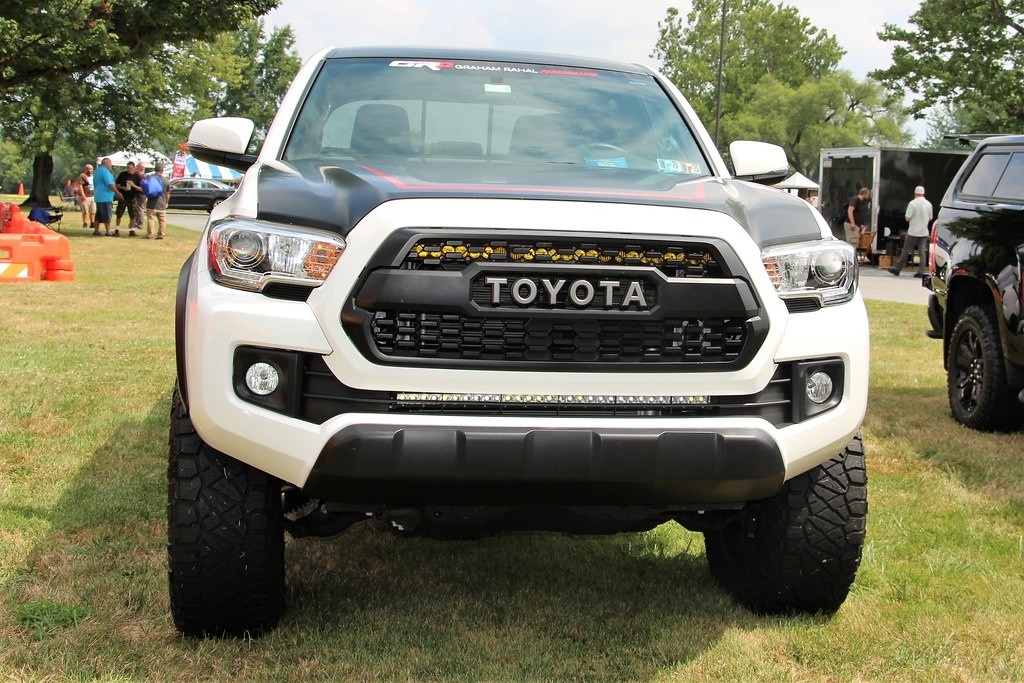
[140,175,163,198]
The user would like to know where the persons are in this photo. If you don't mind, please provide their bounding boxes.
[886,186,933,278]
[844,187,870,265]
[78,163,97,228]
[142,161,171,239]
[111,161,146,237]
[93,158,125,236]
[63,178,82,211]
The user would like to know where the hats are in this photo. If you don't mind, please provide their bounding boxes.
[915,186,925,194]
[154,163,163,171]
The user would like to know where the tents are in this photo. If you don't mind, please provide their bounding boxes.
[96,140,174,177]
[143,155,244,185]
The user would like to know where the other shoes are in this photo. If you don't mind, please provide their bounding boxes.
[130,231,137,236]
[83,223,87,228]
[90,222,95,228]
[115,230,119,237]
[887,268,900,276]
[105,232,115,236]
[93,231,104,236]
[914,273,925,278]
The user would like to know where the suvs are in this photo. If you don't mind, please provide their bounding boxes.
[162,42,876,639]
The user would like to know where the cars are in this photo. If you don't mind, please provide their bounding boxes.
[164,177,235,213]
[925,133,1024,426]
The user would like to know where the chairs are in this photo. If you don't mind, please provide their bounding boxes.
[349,104,413,155]
[856,229,878,266]
[56,185,75,210]
[507,114,569,160]
[32,201,63,232]
